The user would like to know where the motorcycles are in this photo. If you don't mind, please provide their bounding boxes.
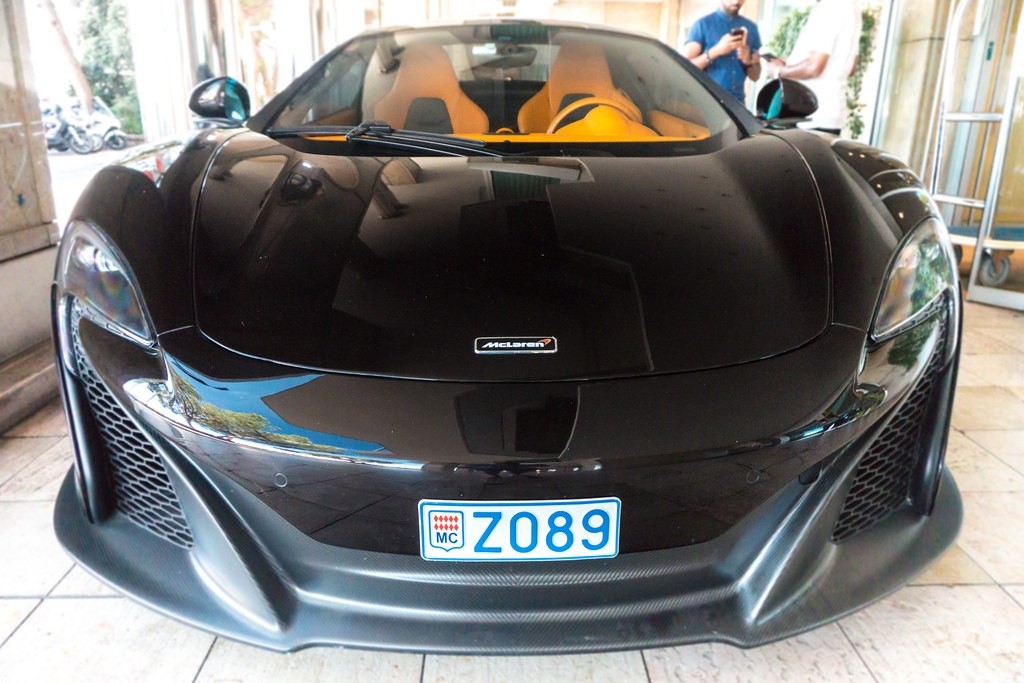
[39,94,130,155]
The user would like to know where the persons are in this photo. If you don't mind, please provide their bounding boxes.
[680,0,761,106]
[766,0,863,136]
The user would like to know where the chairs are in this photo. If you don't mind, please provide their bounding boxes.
[518,39,643,133]
[374,43,490,136]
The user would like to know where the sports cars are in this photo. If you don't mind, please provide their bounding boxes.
[47,16,965,656]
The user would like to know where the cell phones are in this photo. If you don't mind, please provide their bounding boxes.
[731,29,744,41]
